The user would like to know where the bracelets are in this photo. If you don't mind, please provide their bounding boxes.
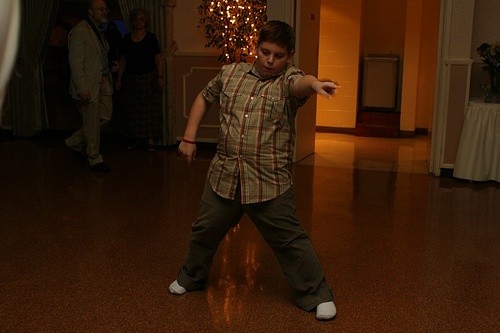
[183,138,196,144]
[158,76,162,78]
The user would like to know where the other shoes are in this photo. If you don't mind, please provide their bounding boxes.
[91,162,110,173]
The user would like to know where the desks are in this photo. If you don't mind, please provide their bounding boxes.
[452,100,500,182]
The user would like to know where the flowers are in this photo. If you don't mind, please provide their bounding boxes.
[475,42,500,95]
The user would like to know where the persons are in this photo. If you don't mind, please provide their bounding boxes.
[64,0,120,175]
[115,8,163,152]
[169,20,342,319]
